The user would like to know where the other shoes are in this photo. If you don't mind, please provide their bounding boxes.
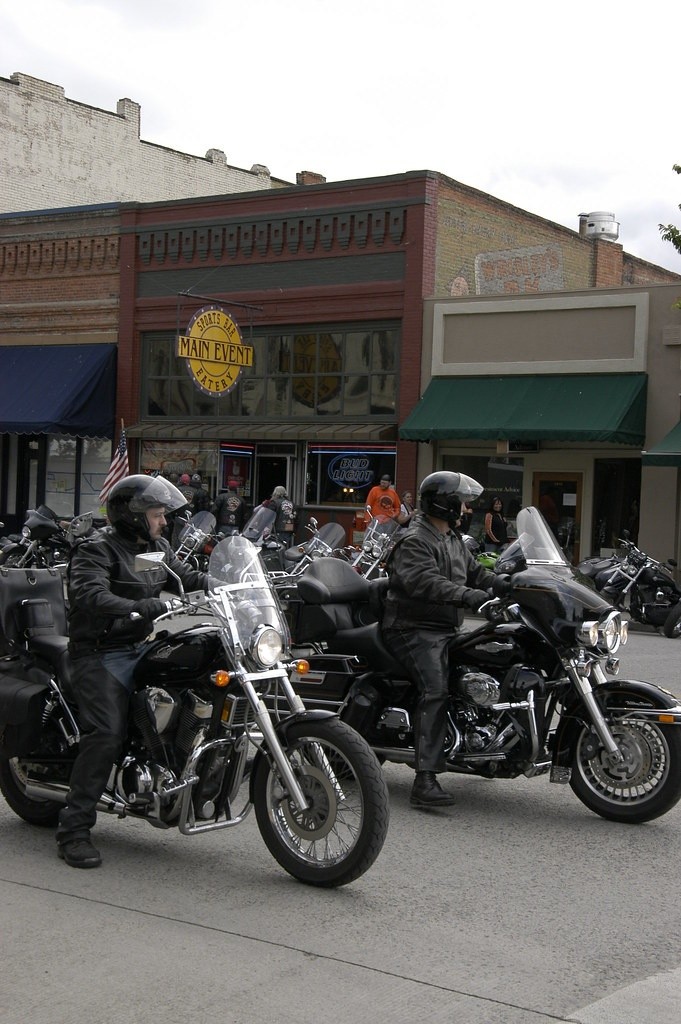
[57,835,102,869]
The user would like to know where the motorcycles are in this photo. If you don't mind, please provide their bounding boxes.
[481,533,626,623]
[0,503,376,623]
[600,537,681,639]
[261,506,681,825]
[351,503,479,583]
[0,536,392,889]
[459,532,509,614]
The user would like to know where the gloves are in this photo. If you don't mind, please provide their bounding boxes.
[132,598,167,621]
[463,589,490,613]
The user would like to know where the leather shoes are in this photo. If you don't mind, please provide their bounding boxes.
[410,769,454,807]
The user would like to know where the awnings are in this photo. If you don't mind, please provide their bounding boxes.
[399,373,648,447]
[0,345,118,441]
[641,420,681,466]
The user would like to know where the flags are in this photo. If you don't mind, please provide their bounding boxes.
[99,430,129,506]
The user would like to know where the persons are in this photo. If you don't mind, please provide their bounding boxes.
[540,489,560,545]
[162,474,210,553]
[365,475,401,524]
[268,486,296,545]
[55,474,228,868]
[485,497,510,552]
[398,491,416,528]
[211,481,247,538]
[383,470,509,805]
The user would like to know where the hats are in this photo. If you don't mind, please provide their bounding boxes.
[228,481,240,486]
[192,474,200,481]
[180,474,190,482]
[402,490,412,497]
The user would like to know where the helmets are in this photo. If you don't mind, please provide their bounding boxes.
[419,471,484,523]
[107,475,187,545]
